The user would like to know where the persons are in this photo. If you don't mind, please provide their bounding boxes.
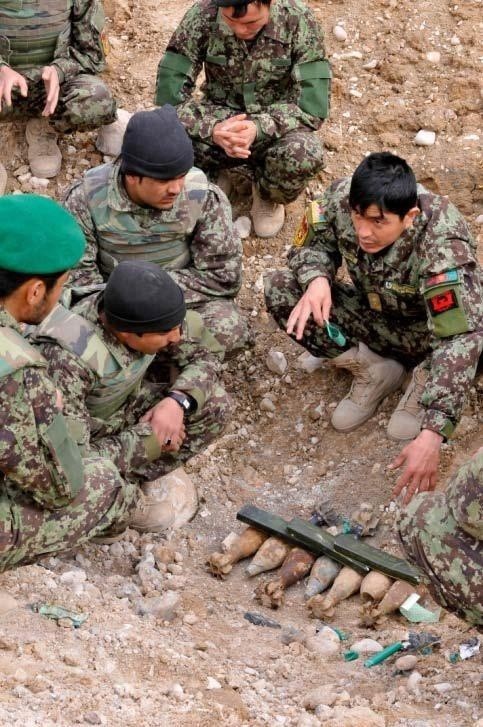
[263,151,483,512]
[153,0,333,237]
[392,443,483,639]
[0,1,118,180]
[27,257,233,533]
[61,107,248,376]
[0,193,129,571]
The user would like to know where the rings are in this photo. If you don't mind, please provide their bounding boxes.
[164,437,172,448]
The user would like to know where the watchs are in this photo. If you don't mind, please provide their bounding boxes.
[165,391,195,410]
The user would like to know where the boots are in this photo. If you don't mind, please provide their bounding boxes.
[26,119,62,178]
[329,342,431,440]
[215,174,284,237]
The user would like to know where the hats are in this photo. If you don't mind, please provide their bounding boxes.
[104,261,185,332]
[122,104,194,179]
[0,194,86,275]
